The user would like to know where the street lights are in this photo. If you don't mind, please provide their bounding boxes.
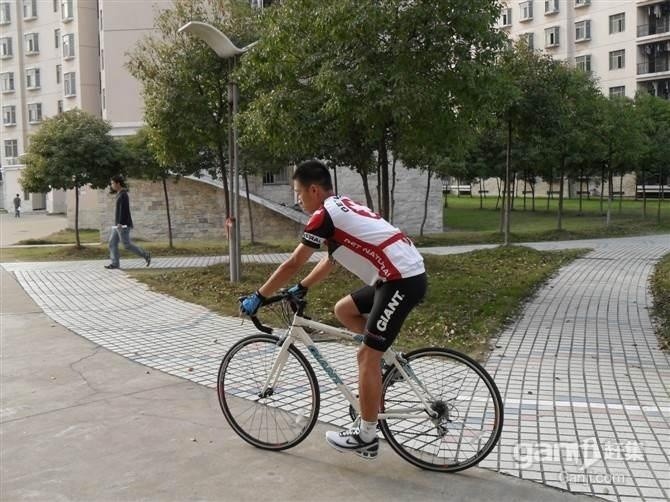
[175,20,263,287]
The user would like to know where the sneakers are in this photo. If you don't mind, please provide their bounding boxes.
[104,263,118,269]
[325,428,379,460]
[144,251,152,266]
[381,365,415,381]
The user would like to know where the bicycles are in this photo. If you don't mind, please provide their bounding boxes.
[215,285,509,476]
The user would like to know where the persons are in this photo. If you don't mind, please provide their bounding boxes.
[103,176,152,270]
[13,194,21,218]
[238,160,428,460]
